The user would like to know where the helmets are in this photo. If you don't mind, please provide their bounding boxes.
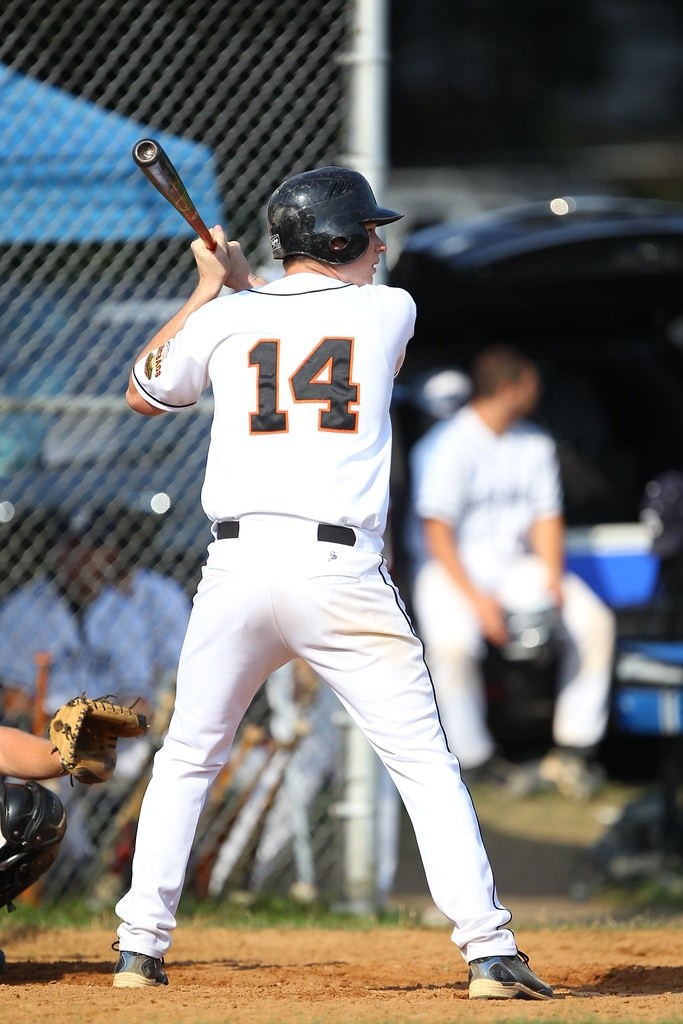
[264,166,402,264]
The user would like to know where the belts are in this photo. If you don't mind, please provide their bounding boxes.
[217,520,356,547]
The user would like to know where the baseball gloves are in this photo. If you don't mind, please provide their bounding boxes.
[48,691,151,787]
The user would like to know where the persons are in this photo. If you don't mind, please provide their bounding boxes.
[0,525,153,904]
[108,528,192,691]
[406,346,616,801]
[109,172,557,1001]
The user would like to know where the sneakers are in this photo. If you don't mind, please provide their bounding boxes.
[110,952,169,987]
[472,744,600,803]
[467,954,556,999]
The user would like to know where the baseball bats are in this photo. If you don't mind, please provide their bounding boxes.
[130,136,220,252]
